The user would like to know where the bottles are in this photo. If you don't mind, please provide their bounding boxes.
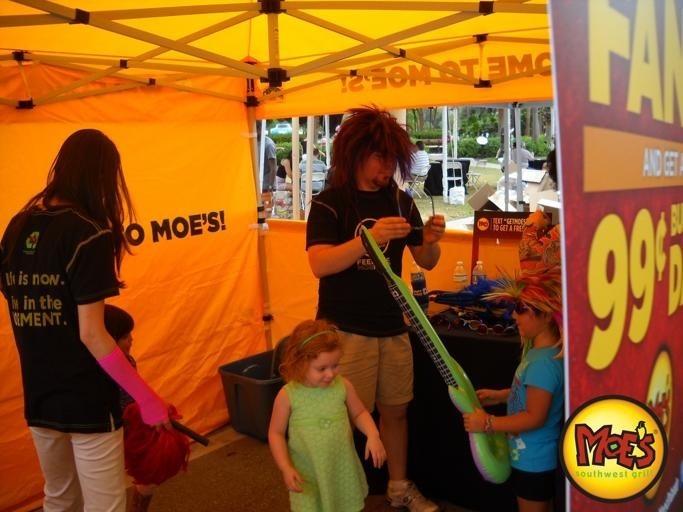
[409,260,428,314]
[472,260,487,285]
[452,262,467,292]
[518,201,530,213]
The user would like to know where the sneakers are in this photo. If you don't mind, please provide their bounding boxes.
[386,480,439,512]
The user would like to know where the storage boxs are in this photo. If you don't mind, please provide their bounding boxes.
[218,349,290,442]
[467,167,560,230]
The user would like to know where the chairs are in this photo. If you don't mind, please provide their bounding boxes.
[441,162,465,201]
[300,171,326,210]
[407,165,431,198]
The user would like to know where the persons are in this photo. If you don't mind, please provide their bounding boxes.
[104,304,153,512]
[255,121,555,221]
[462,255,566,512]
[0,128,178,512]
[304,104,439,512]
[267,319,387,512]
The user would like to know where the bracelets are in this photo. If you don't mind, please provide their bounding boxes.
[484,414,497,435]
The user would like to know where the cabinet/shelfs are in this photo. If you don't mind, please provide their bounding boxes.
[409,307,525,506]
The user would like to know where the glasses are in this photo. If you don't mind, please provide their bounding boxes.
[508,304,527,314]
[458,318,515,336]
